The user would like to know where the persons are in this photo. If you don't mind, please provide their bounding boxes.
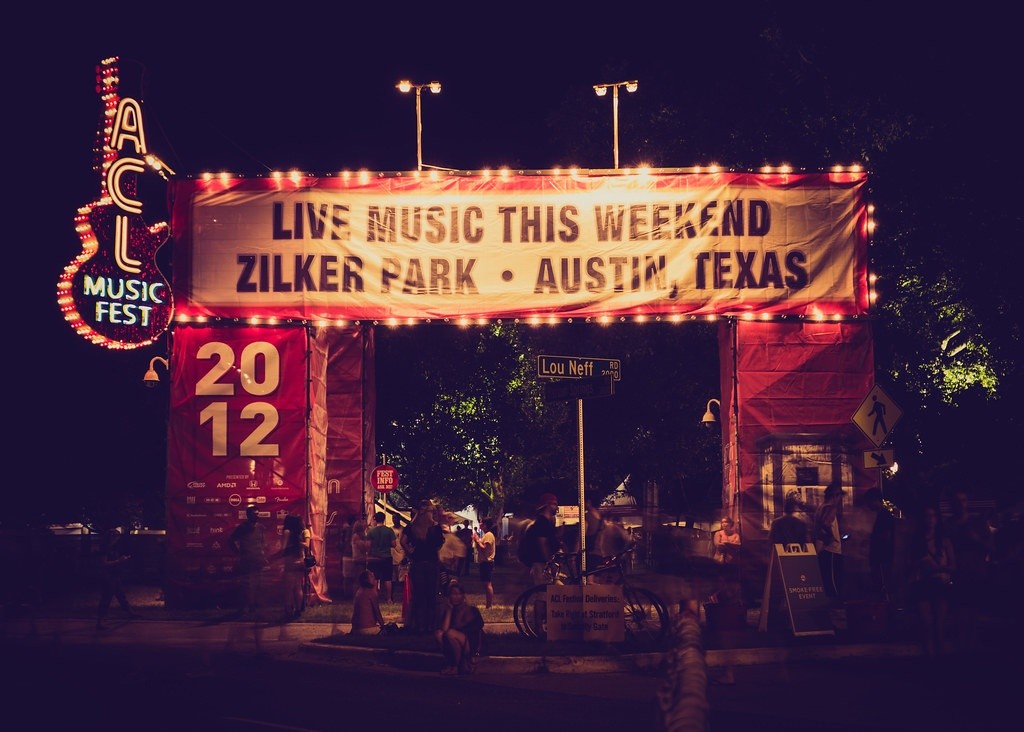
[229,508,306,620]
[865,486,1024,652]
[577,494,741,583]
[96,530,143,633]
[769,487,843,600]
[338,500,498,676]
[529,495,560,641]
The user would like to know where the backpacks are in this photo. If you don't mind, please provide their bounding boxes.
[517,520,547,567]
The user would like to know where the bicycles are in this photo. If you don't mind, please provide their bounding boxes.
[513,546,670,645]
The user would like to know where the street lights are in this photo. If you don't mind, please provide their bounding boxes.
[593,80,641,169]
[396,78,443,172]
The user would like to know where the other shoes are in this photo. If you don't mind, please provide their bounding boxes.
[440,669,460,678]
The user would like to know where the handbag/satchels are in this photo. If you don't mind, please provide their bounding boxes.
[304,556,316,568]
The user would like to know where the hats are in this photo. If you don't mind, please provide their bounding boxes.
[536,493,558,510]
